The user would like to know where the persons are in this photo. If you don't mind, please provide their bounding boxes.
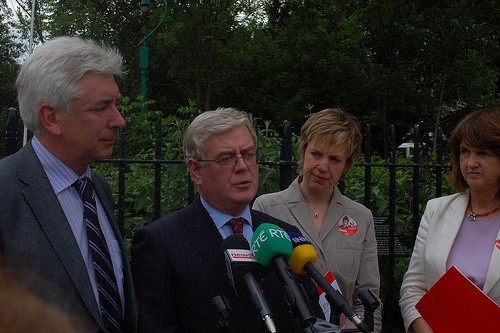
[340,216,349,227]
[251,107,382,333]
[0,37,137,333]
[399,106,500,333]
[130,108,326,333]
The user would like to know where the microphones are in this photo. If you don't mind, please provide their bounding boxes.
[250,217,319,333]
[286,232,372,333]
[220,234,278,333]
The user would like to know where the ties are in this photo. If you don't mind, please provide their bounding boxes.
[72,178,124,333]
[225,218,246,235]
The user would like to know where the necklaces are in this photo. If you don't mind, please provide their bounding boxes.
[306,203,326,218]
[467,198,500,221]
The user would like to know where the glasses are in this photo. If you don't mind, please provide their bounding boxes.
[199,152,260,169]
